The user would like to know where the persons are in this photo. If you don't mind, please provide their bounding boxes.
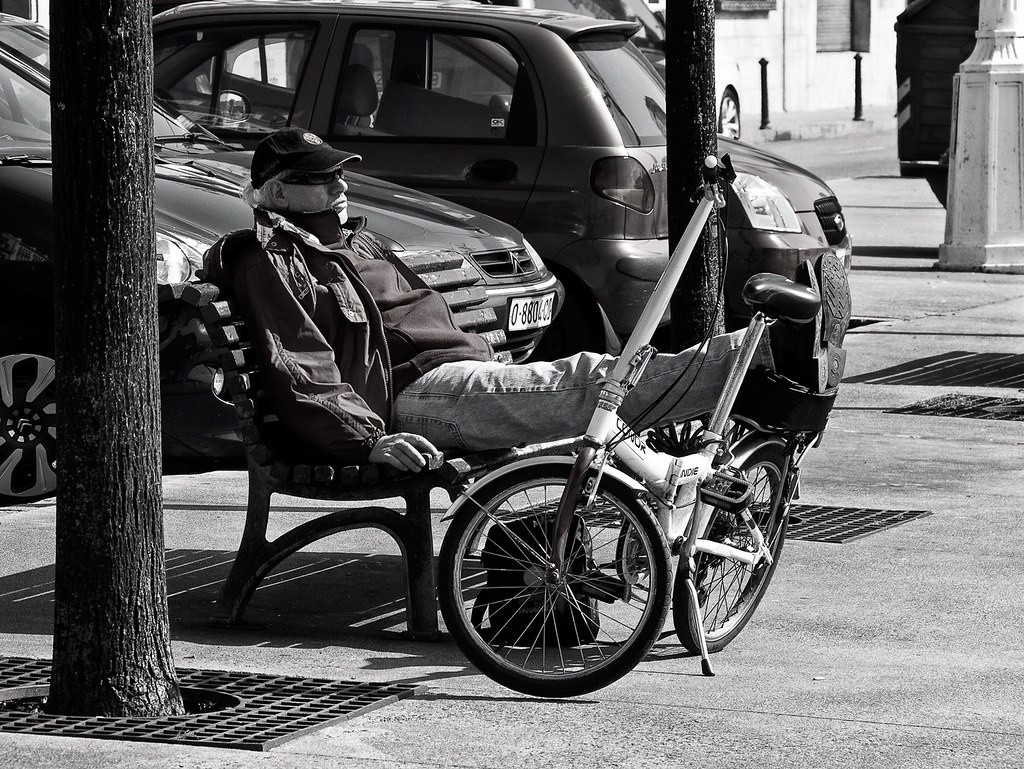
[225,128,854,473]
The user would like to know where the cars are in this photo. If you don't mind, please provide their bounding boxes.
[153,0,852,336]
[0,12,565,502]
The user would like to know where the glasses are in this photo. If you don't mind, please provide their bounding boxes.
[280,167,345,186]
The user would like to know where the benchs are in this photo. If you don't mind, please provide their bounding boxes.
[159,247,706,645]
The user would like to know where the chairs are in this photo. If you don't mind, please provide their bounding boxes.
[338,64,379,125]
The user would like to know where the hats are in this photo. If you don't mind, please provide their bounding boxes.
[251,127,362,191]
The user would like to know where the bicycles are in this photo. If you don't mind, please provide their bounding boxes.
[437,153,838,696]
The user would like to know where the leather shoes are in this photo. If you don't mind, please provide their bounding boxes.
[769,258,829,394]
[814,251,852,390]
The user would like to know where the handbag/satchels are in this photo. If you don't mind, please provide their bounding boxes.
[471,514,601,648]
